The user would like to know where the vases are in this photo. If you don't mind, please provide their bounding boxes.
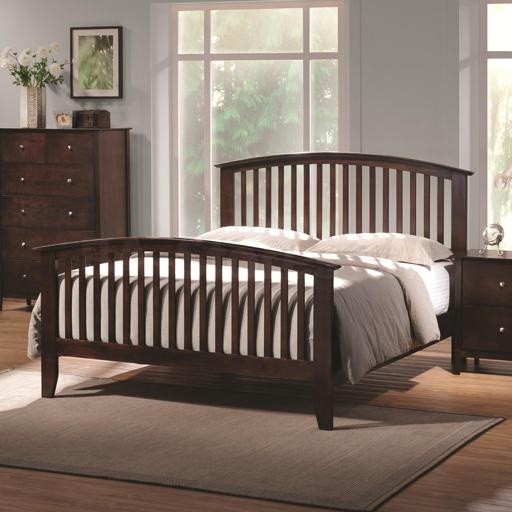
[19,86,45,128]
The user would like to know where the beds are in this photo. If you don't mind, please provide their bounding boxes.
[28,153,474,430]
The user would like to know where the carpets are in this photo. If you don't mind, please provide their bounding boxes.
[0,378,505,511]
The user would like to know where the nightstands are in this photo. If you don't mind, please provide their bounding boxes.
[449,250,512,376]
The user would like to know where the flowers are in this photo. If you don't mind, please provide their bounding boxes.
[0,40,69,87]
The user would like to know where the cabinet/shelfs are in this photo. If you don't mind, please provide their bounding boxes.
[1,128,131,310]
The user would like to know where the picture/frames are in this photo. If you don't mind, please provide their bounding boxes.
[69,26,122,98]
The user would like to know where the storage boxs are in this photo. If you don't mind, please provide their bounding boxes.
[72,110,110,127]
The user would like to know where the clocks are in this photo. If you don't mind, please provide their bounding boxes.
[479,223,504,255]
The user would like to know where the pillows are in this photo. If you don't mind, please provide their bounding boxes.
[183,225,318,273]
[305,231,452,269]
